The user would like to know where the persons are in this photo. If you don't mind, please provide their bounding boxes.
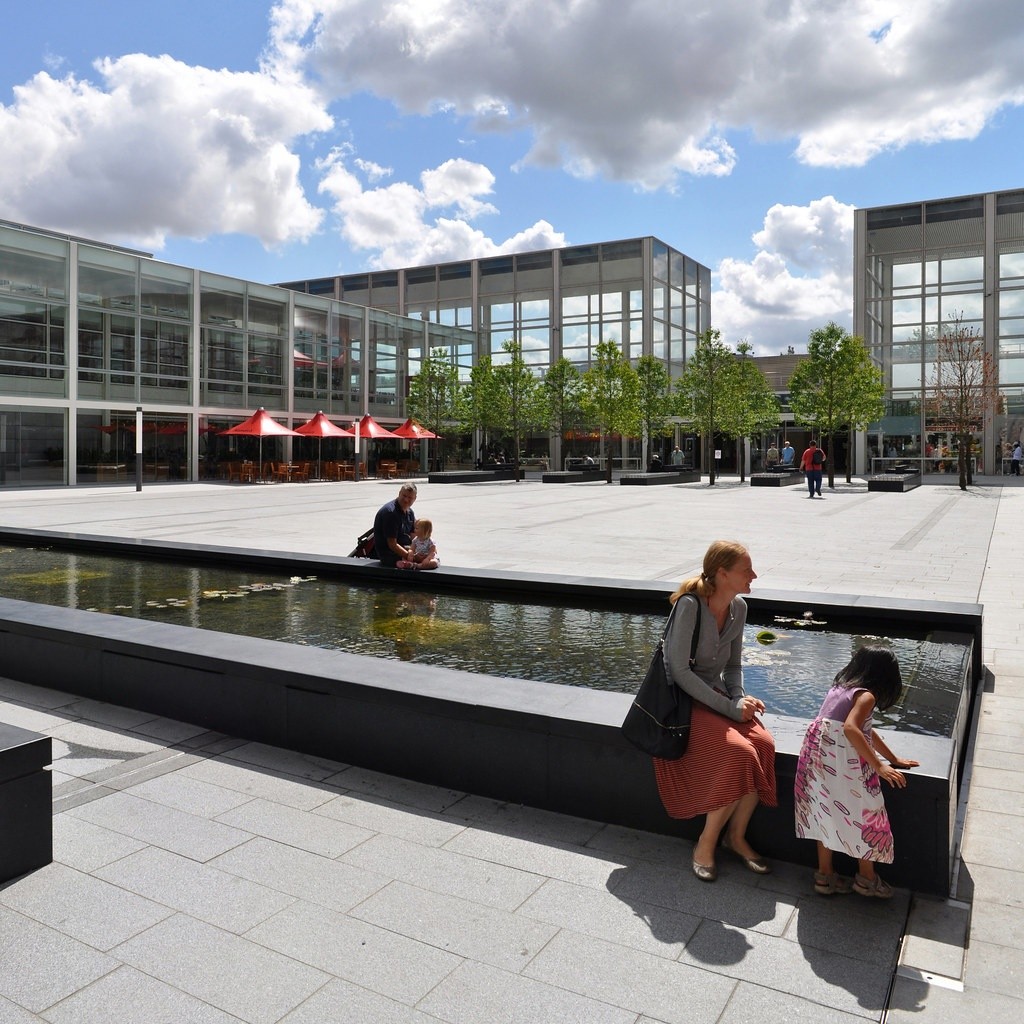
[582,455,594,465]
[1010,441,1022,476]
[671,446,685,464]
[485,453,506,464]
[374,482,417,567]
[799,439,826,498]
[651,539,778,882]
[782,440,795,464]
[766,443,779,465]
[564,451,571,471]
[396,518,441,569]
[794,645,920,897]
[650,454,662,472]
[540,452,549,471]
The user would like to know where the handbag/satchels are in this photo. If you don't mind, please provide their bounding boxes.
[812,448,824,465]
[618,594,703,760]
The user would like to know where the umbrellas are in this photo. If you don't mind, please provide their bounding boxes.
[215,407,306,482]
[391,418,446,477]
[293,411,356,482]
[95,419,240,453]
[248,347,374,374]
[346,412,405,478]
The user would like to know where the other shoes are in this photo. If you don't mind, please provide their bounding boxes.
[396,560,418,570]
[690,841,716,881]
[816,489,821,496]
[810,494,814,498]
[721,840,771,874]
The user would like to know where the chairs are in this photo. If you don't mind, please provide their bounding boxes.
[228,459,420,484]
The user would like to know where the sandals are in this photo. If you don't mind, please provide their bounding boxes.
[852,871,895,897]
[815,870,854,894]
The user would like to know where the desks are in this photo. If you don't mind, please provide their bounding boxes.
[237,464,258,484]
[337,464,356,480]
[381,463,398,478]
[280,465,298,483]
